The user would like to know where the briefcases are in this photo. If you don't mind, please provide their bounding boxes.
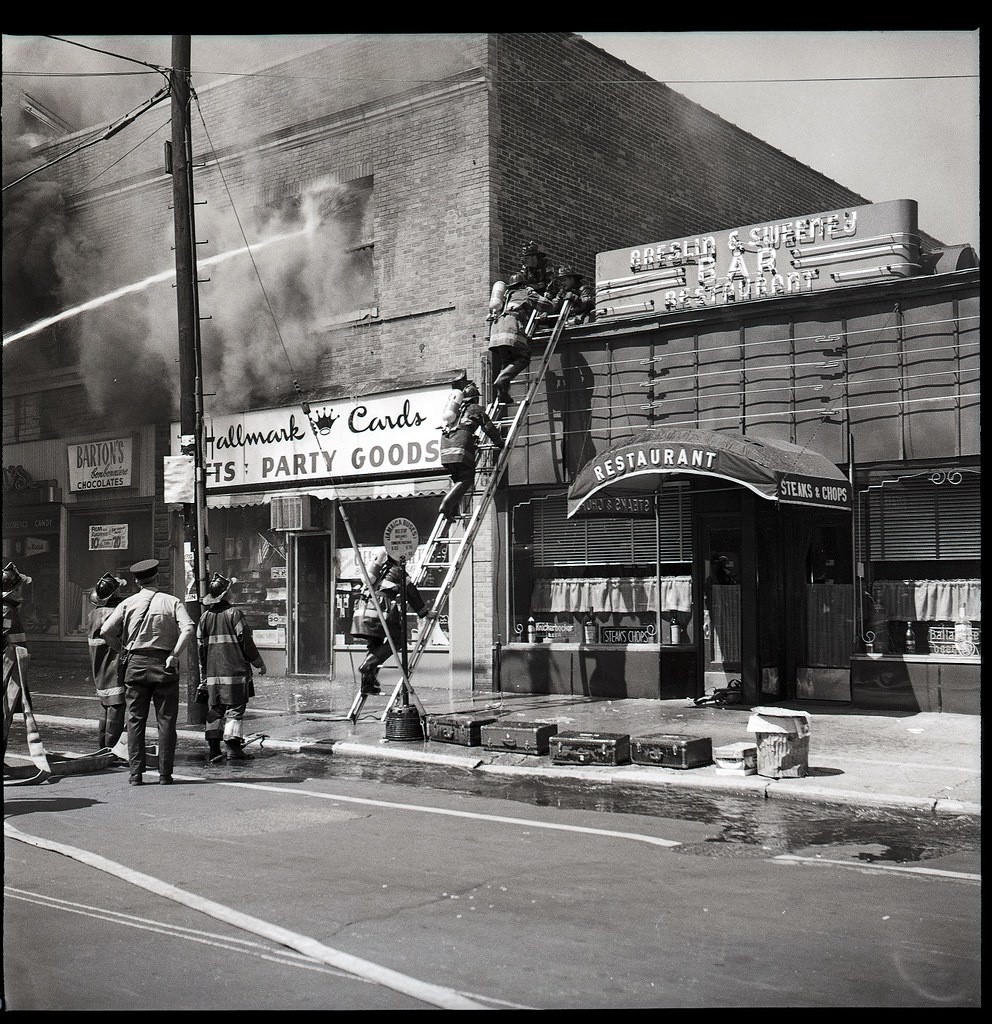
[480,720,558,756]
[630,733,713,769]
[427,714,498,747]
[549,730,631,766]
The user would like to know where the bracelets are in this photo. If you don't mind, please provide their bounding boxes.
[169,650,180,657]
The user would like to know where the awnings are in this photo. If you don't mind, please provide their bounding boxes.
[566,427,855,521]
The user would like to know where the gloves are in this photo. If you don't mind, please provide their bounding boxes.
[563,291,578,303]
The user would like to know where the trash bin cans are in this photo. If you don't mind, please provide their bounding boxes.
[750,705,810,779]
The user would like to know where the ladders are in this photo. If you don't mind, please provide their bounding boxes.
[346,291,577,724]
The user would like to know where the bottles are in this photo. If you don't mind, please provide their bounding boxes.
[670,610,680,645]
[954,603,972,655]
[905,622,915,653]
[584,606,598,644]
[871,588,888,654]
[527,607,536,643]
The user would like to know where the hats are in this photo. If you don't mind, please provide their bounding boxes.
[130,559,159,581]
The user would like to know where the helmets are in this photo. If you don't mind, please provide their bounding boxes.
[518,241,547,258]
[462,384,483,401]
[89,572,127,606]
[2,562,32,598]
[557,264,586,280]
[505,272,529,287]
[202,572,237,605]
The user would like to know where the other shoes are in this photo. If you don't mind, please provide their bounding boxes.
[493,380,514,404]
[129,772,143,786]
[439,503,456,523]
[159,775,173,784]
[358,665,380,686]
[209,739,227,763]
[224,740,255,767]
[361,678,381,694]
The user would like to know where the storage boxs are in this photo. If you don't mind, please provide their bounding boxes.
[712,741,757,778]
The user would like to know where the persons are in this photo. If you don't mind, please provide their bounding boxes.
[438,383,514,523]
[87,571,127,751]
[99,558,195,786]
[0,561,32,780]
[196,572,267,765]
[349,550,439,698]
[513,241,593,327]
[487,271,554,404]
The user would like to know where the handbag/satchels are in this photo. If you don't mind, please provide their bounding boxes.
[194,683,209,705]
[117,652,128,686]
